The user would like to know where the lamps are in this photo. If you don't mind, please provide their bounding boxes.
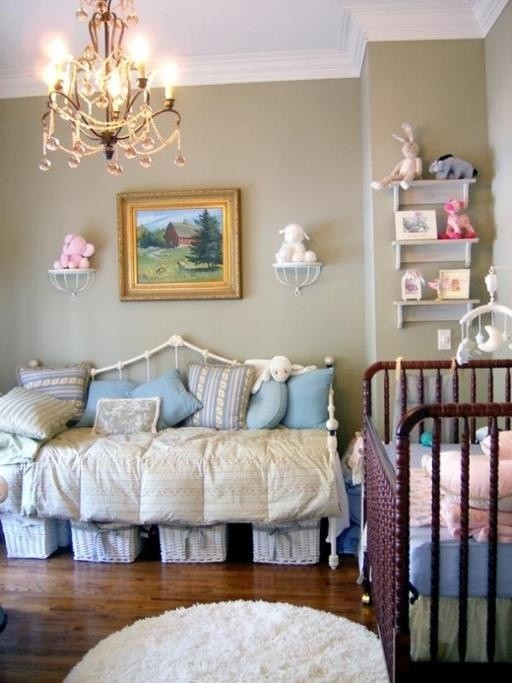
[35,0,186,176]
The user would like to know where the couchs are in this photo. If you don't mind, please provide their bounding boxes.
[0,332,339,571]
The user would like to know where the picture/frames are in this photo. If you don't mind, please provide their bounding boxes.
[394,209,437,240]
[437,269,470,299]
[401,268,426,301]
[115,187,242,301]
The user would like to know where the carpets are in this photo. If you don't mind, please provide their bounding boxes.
[59,599,390,683]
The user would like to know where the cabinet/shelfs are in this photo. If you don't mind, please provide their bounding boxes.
[389,178,481,329]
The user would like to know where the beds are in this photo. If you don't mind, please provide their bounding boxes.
[361,358,512,683]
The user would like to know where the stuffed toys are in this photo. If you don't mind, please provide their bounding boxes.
[275,223,317,264]
[428,153,478,180]
[438,197,476,240]
[251,355,317,395]
[370,120,424,192]
[52,233,96,270]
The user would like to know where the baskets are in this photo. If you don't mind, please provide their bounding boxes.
[156,523,227,566]
[251,517,323,567]
[71,520,142,564]
[1,513,70,561]
